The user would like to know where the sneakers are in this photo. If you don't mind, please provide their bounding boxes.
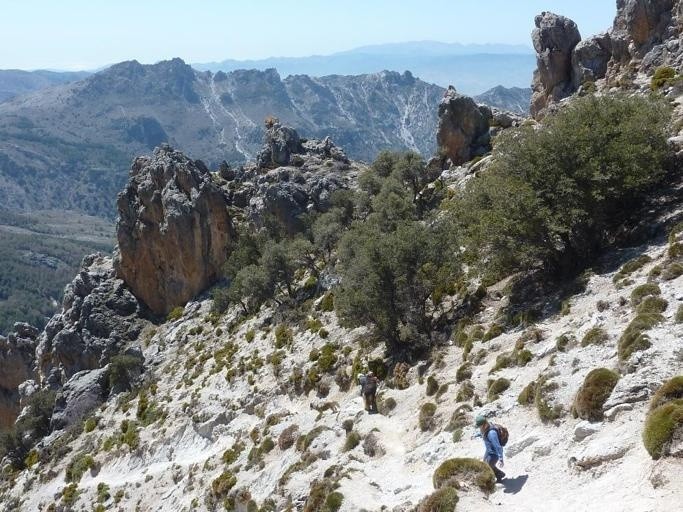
[496,471,505,483]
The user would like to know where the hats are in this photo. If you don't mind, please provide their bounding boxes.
[474,414,485,428]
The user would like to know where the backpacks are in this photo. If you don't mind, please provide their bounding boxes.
[485,424,509,446]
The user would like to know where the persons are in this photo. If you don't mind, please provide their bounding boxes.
[470,415,504,483]
[365,371,379,414]
[357,373,368,410]
